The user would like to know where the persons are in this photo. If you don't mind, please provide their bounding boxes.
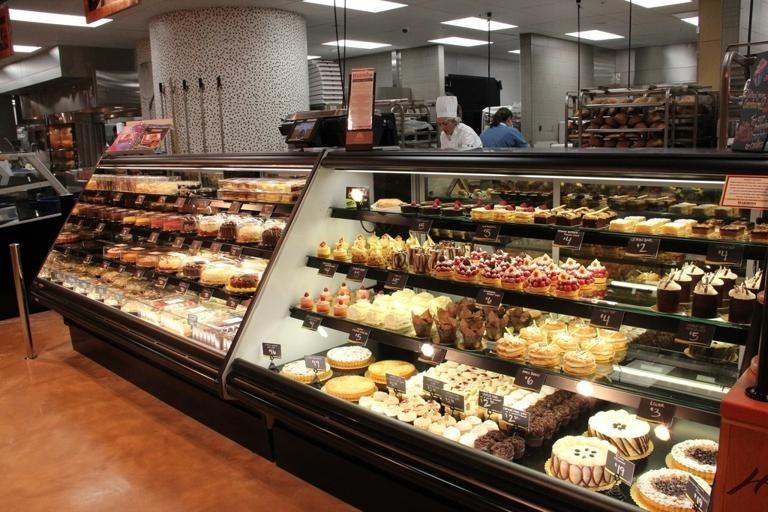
[435,95,483,149]
[480,107,528,148]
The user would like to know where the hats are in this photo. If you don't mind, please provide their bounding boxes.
[435,95,458,120]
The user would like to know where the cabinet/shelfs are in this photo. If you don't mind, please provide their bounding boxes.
[581,86,698,148]
[719,51,767,151]
[220,148,768,512]
[28,150,327,427]
[392,102,435,147]
[563,90,582,149]
[0,152,74,322]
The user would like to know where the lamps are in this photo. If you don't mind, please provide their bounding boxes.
[350,188,378,232]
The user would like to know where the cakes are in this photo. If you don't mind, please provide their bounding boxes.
[326,346,375,370]
[401,199,485,217]
[183,211,287,249]
[433,246,607,297]
[365,360,419,385]
[666,438,719,485]
[545,435,619,491]
[630,467,712,511]
[226,273,258,291]
[183,261,208,277]
[412,296,531,350]
[608,215,698,236]
[497,308,628,375]
[587,409,654,459]
[534,204,617,229]
[318,233,436,265]
[75,202,182,235]
[321,375,379,402]
[391,241,471,273]
[103,242,183,272]
[280,360,334,383]
[419,362,587,447]
[669,202,739,220]
[359,385,527,461]
[691,218,768,241]
[300,283,385,318]
[657,261,762,323]
[348,288,451,330]
[200,264,234,284]
[470,200,549,223]
